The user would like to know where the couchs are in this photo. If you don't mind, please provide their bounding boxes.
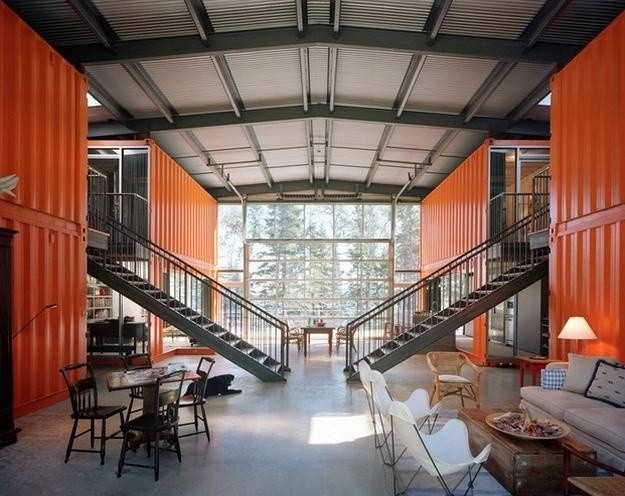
[519,362,625,478]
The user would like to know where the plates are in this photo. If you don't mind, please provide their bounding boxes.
[485,411,571,440]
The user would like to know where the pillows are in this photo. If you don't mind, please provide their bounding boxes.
[540,352,625,407]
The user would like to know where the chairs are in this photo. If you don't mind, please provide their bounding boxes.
[357,359,492,496]
[279,318,304,352]
[336,321,357,353]
[561,441,625,496]
[58,354,215,481]
[425,351,482,408]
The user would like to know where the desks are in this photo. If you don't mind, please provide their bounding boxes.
[86,322,145,355]
[515,355,558,399]
[299,324,335,357]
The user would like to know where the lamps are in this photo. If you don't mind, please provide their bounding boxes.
[12,303,58,342]
[557,316,598,353]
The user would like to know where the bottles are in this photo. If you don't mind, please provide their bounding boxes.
[306,316,326,327]
